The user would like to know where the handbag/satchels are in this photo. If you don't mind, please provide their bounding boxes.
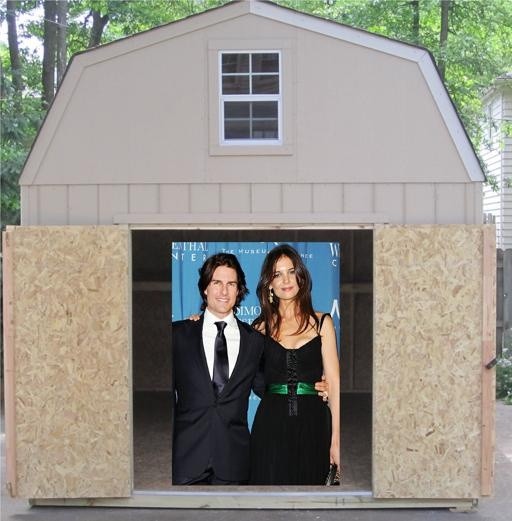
[324,464,340,485]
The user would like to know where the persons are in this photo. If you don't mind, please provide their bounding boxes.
[187,244,340,486]
[172,252,330,485]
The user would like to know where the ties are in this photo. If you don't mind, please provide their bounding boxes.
[212,321,229,397]
